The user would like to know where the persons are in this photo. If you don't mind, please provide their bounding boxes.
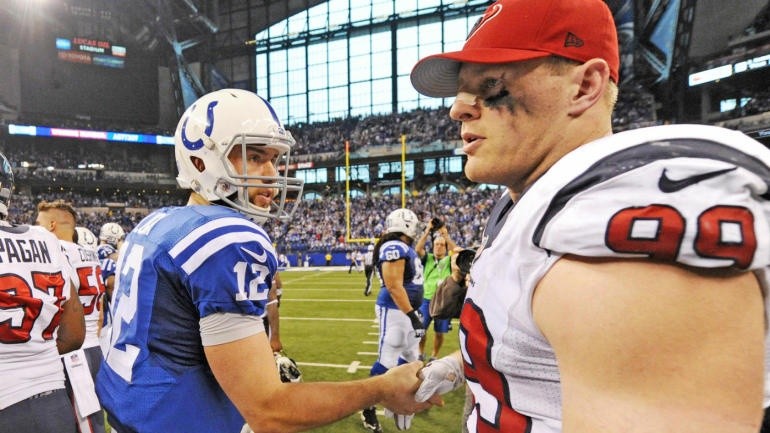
[362,244,374,296]
[262,184,506,273]
[97,222,125,263]
[0,145,189,246]
[34,199,106,433]
[278,104,461,156]
[731,94,770,117]
[0,148,85,433]
[93,88,446,433]
[415,216,458,365]
[393,0,770,432]
[73,226,115,357]
[361,208,425,433]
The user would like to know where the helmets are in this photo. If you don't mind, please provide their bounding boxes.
[99,222,125,245]
[174,88,296,202]
[386,208,417,240]
[0,154,15,216]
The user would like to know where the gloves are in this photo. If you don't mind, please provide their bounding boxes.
[384,349,466,431]
[407,310,426,337]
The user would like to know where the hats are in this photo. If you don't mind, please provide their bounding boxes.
[411,0,620,98]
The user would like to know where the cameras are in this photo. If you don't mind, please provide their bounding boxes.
[432,217,443,228]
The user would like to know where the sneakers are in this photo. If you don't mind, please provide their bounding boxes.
[360,408,381,432]
